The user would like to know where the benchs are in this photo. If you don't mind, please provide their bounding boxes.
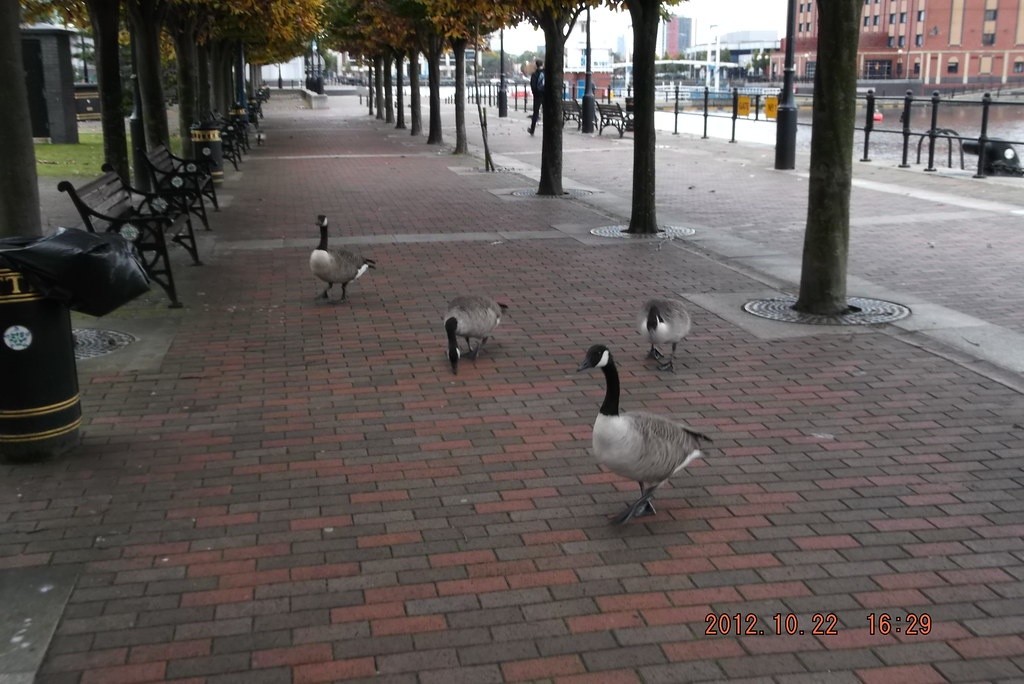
[143,144,220,230]
[57,164,201,310]
[561,97,600,131]
[196,86,269,171]
[595,101,635,140]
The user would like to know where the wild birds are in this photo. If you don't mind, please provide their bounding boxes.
[635,293,693,371]
[444,294,509,376]
[310,214,376,305]
[576,344,715,525]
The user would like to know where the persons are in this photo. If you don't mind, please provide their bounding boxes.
[527,60,545,136]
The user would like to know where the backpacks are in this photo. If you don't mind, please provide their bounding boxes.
[537,71,544,90]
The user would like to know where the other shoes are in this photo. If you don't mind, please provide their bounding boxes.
[528,127,533,134]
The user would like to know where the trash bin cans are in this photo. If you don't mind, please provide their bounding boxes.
[190,129,225,185]
[1,237,87,464]
[237,107,249,126]
[626,97,634,131]
[74,92,102,120]
[765,97,778,118]
[738,95,750,115]
